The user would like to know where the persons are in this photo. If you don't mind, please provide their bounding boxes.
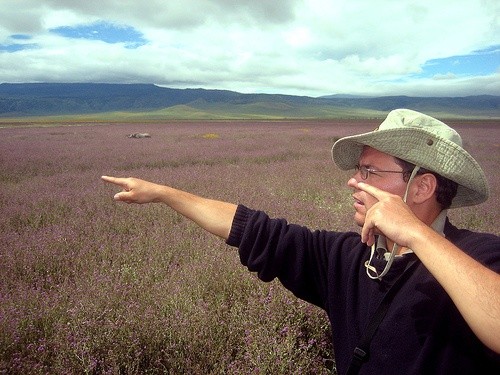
[101,108,500,375]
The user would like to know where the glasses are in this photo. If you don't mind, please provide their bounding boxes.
[354,164,412,180]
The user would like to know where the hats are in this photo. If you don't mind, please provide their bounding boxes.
[331,108,491,210]
